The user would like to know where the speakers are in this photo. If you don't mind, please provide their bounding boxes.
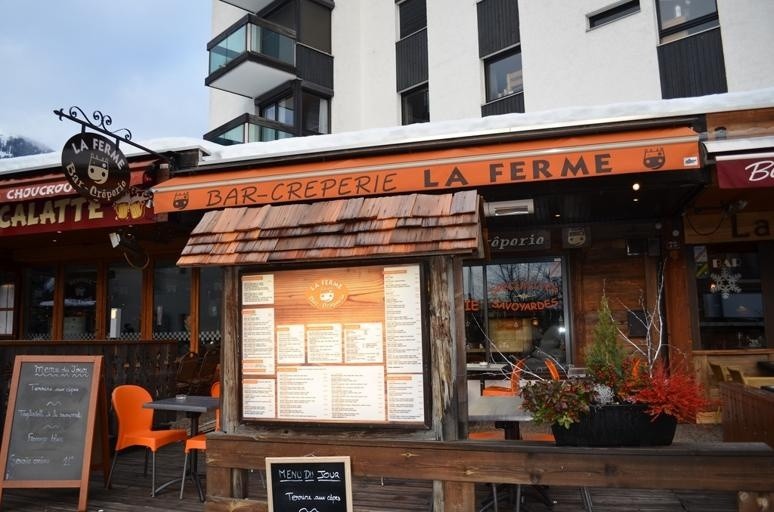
[702,293,721,321]
[627,310,647,337]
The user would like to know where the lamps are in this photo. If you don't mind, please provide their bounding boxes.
[108,228,143,257]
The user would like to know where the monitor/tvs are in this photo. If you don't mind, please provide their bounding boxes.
[721,290,764,322]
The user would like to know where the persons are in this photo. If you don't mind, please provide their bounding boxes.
[523,309,566,367]
[174,314,191,364]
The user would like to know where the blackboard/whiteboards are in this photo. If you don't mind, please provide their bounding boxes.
[265,456,353,512]
[0,355,103,488]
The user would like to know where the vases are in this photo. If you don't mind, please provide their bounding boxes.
[544,404,676,448]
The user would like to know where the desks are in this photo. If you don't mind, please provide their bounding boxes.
[467,361,509,397]
[143,396,220,503]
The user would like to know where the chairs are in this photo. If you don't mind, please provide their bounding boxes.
[484,357,644,397]
[174,350,220,392]
[106,383,187,498]
[183,382,221,499]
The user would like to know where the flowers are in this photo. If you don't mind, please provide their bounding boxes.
[516,362,711,433]
